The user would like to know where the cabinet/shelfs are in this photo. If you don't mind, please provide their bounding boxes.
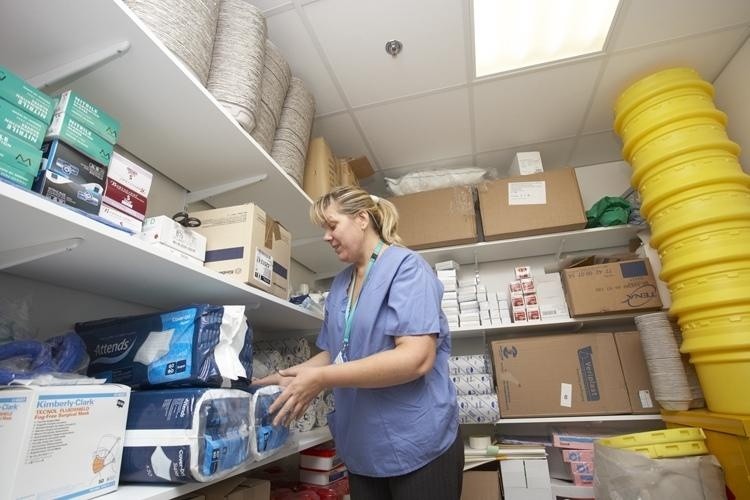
[412,224,662,499]
[1,0,354,500]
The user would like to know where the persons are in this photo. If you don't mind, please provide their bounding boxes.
[250,185,465,500]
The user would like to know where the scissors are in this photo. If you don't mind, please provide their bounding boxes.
[172,210,201,228]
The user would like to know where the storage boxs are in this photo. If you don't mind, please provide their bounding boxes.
[476,166,588,240]
[0,161,34,190]
[449,373,496,395]
[141,214,208,262]
[39,138,107,196]
[560,257,663,318]
[572,474,593,485]
[500,459,553,500]
[551,431,625,449]
[455,394,500,424]
[0,99,48,149]
[560,449,595,462]
[0,379,132,499]
[338,156,376,189]
[614,328,663,415]
[460,464,503,500]
[0,128,43,177]
[490,332,632,419]
[44,112,113,168]
[448,353,494,374]
[569,462,594,474]
[31,169,102,216]
[383,184,477,251]
[104,151,153,198]
[53,90,123,146]
[98,202,143,235]
[0,63,56,125]
[188,202,291,299]
[102,176,147,222]
[303,136,341,204]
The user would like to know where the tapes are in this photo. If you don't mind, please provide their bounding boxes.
[469,434,492,451]
